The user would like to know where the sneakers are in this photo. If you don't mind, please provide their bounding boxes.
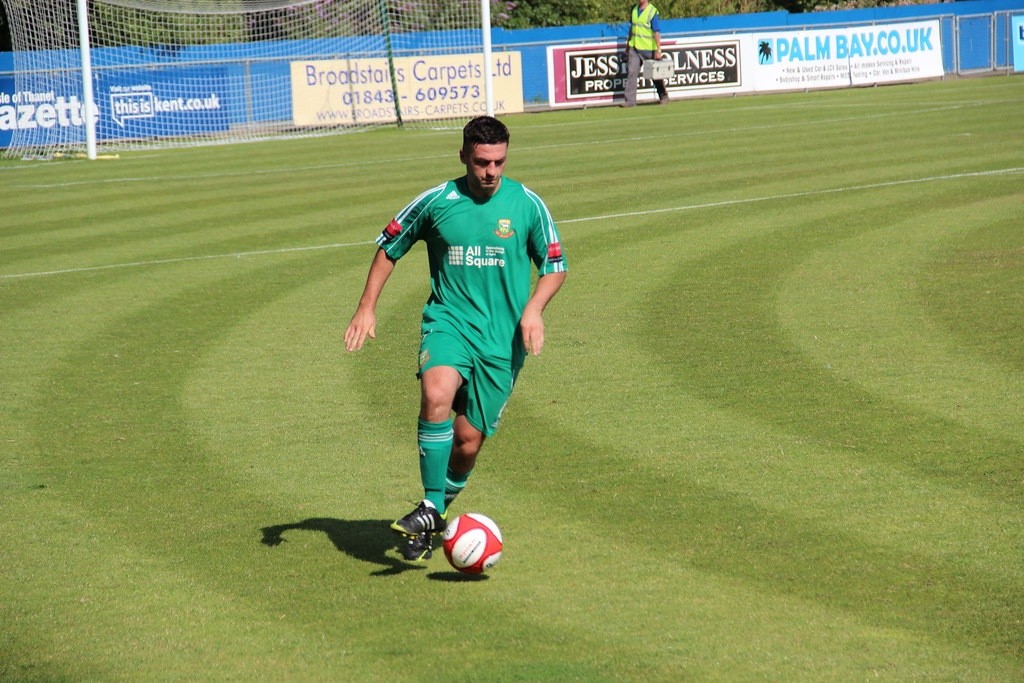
[384,499,449,562]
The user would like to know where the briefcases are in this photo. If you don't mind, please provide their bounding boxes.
[642,58,674,79]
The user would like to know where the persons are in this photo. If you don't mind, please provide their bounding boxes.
[620,0,670,107]
[342,115,568,567]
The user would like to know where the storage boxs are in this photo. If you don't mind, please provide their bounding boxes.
[642,58,675,80]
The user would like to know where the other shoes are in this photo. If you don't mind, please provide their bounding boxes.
[620,94,669,106]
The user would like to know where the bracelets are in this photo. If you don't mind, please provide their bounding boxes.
[656,48,662,53]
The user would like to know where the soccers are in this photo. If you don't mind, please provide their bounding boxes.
[444,514,504,575]
